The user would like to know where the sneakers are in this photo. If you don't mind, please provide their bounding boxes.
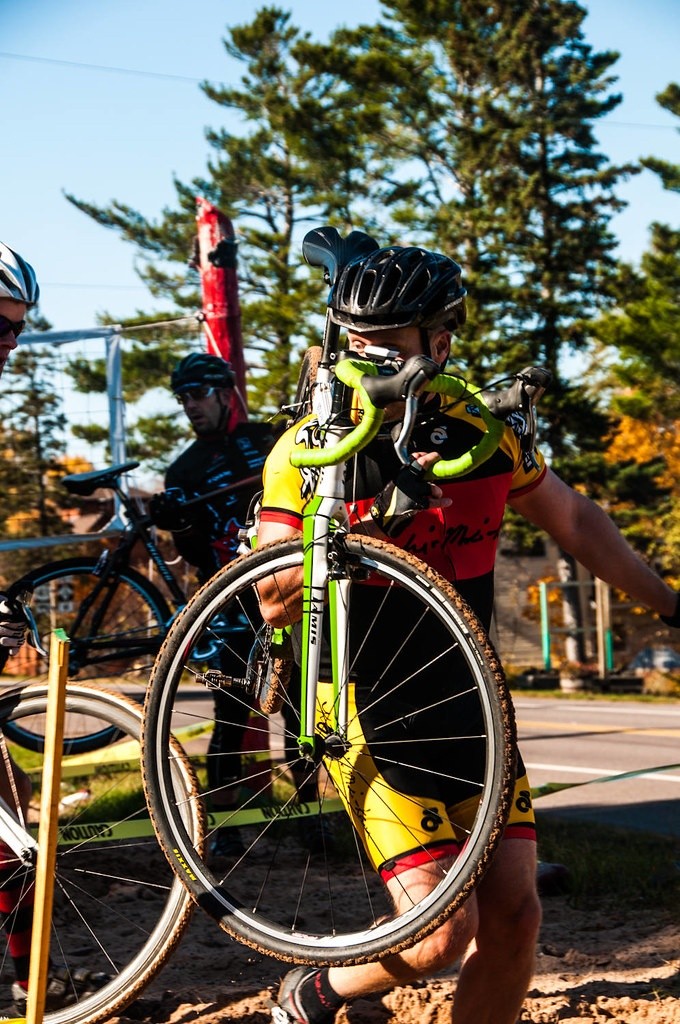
[11,963,121,1014]
[270,964,323,1024]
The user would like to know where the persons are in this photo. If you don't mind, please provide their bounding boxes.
[0,241,116,1024]
[148,351,326,855]
[253,246,680,1024]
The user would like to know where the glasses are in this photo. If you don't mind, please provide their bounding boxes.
[0,314,26,338]
[176,385,228,405]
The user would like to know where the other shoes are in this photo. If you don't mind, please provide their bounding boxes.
[296,817,335,854]
[209,828,245,857]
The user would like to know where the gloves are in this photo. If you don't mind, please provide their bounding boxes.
[0,594,27,656]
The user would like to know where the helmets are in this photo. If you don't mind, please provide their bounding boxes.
[0,241,40,310]
[169,352,236,394]
[328,246,467,332]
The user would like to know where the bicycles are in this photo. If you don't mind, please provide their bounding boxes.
[0,591,210,1023]
[139,223,555,969]
[0,401,306,756]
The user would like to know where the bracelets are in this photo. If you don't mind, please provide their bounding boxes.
[659,592,680,628]
